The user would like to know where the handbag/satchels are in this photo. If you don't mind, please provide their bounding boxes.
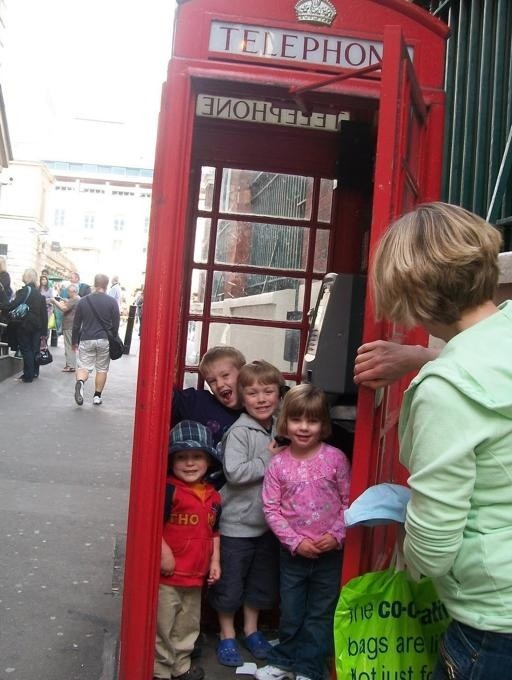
[49,313,58,330]
[35,348,53,365]
[8,303,30,323]
[109,334,126,360]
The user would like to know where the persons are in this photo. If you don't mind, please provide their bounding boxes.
[0,255,145,405]
[354,202,512,680]
[153,419,223,680]
[254,383,350,680]
[215,360,289,666]
[171,346,246,659]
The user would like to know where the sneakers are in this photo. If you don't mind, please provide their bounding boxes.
[15,375,33,384]
[173,665,205,680]
[253,664,296,680]
[92,395,104,404]
[62,366,76,372]
[75,380,84,405]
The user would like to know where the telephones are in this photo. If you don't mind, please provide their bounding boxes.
[274,385,292,445]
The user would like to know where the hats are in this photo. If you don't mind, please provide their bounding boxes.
[167,420,223,462]
[340,481,410,526]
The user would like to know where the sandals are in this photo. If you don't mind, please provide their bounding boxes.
[217,634,244,666]
[238,629,274,659]
